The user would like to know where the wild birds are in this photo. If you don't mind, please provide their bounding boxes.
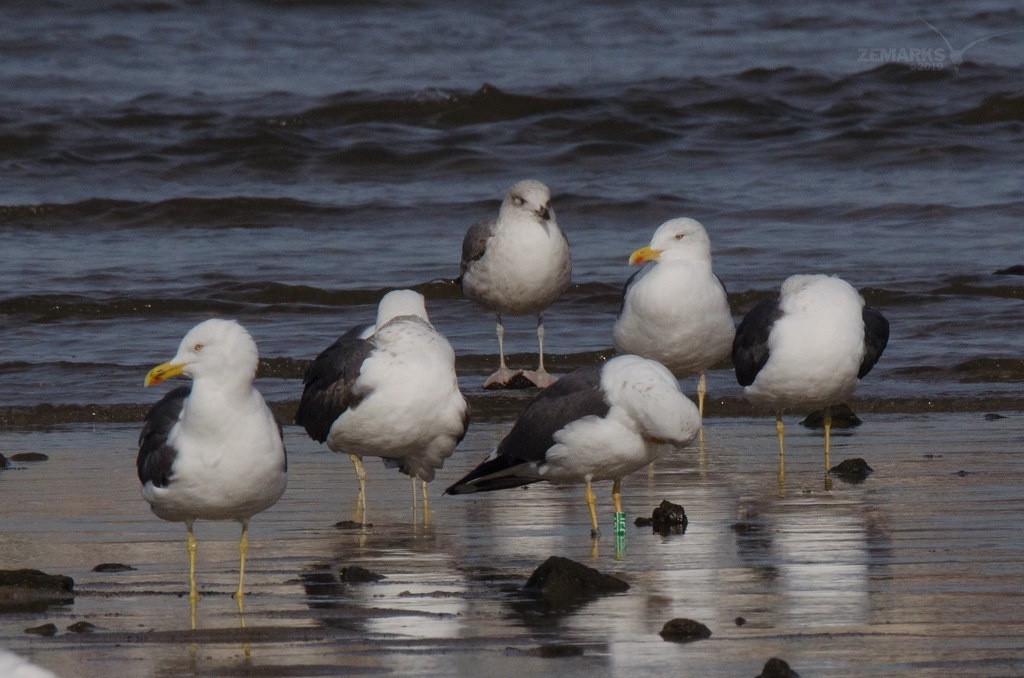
[294,181,892,562]
[137,318,287,614]
